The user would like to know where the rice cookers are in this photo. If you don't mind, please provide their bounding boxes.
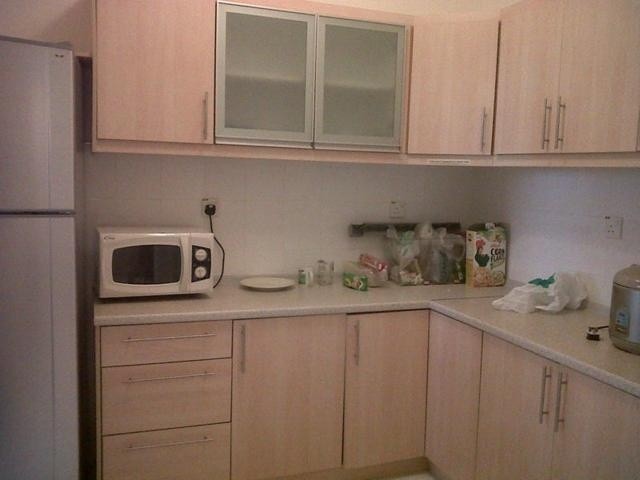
[608,261,640,356]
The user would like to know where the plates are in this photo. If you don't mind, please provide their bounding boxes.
[237,275,296,293]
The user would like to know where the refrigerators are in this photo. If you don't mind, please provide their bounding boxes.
[0,32,87,477]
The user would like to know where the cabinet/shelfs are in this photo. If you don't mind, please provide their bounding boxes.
[406,13,500,168]
[476,329,640,479]
[426,309,484,480]
[232,313,345,480]
[344,309,429,480]
[91,0,216,160]
[493,0,639,169]
[215,1,413,170]
[94,318,232,480]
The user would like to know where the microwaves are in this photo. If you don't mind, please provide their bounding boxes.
[92,223,215,299]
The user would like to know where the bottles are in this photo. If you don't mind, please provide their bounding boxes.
[297,260,337,288]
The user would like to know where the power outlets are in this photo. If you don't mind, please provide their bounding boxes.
[200,195,223,219]
[604,215,624,239]
